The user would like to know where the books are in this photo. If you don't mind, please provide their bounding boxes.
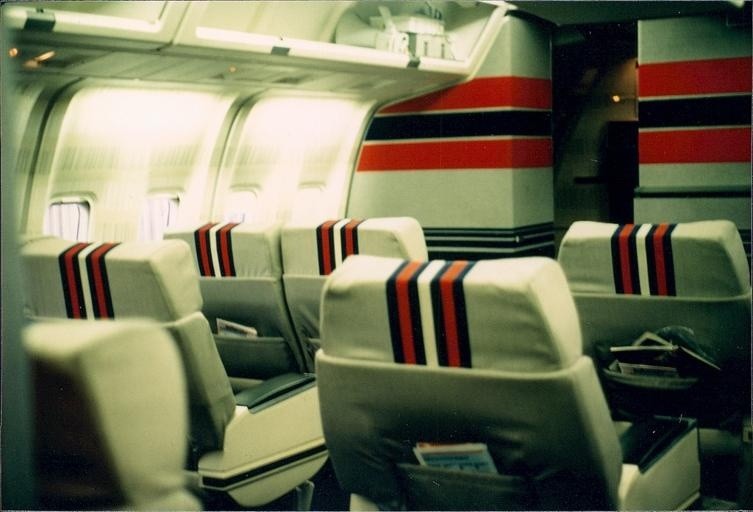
[607,331,721,379]
[216,318,257,338]
[412,442,499,476]
